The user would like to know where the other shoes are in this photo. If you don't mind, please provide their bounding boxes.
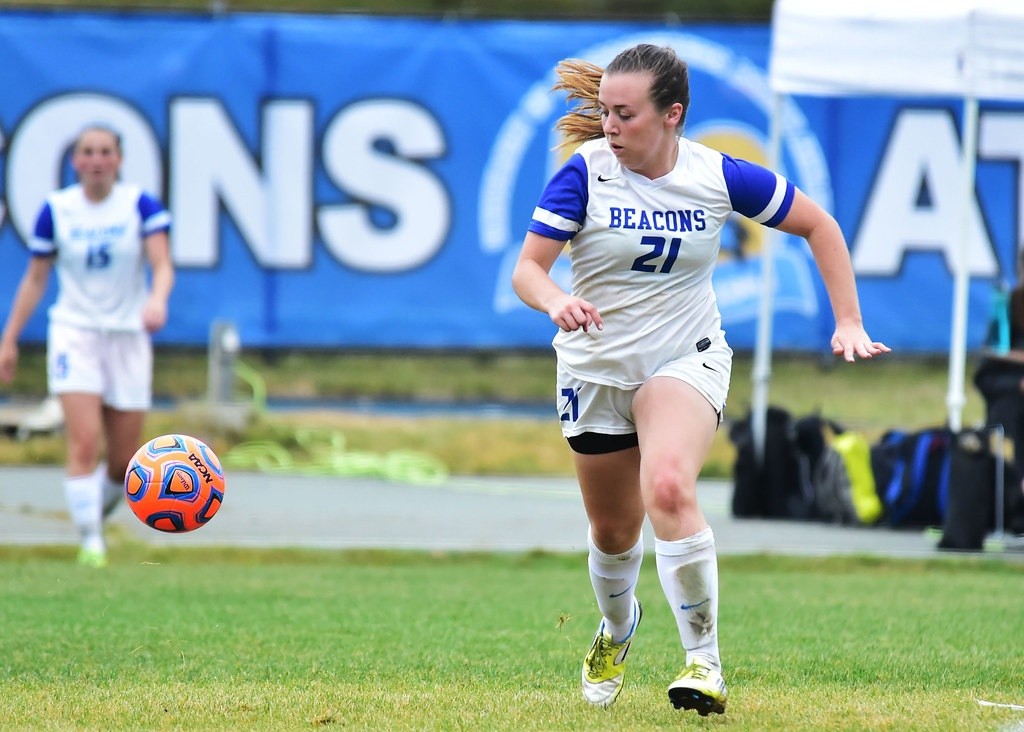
[79,541,108,570]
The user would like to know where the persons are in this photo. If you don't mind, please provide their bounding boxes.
[508,39,893,718]
[2,121,177,574]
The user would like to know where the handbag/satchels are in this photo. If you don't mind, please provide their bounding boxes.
[729,405,1023,552]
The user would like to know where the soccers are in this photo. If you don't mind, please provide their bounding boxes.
[123,435,224,533]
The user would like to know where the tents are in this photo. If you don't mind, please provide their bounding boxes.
[749,0,1024,476]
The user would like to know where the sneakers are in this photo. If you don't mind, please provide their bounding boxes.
[667,658,728,716]
[582,602,644,708]
